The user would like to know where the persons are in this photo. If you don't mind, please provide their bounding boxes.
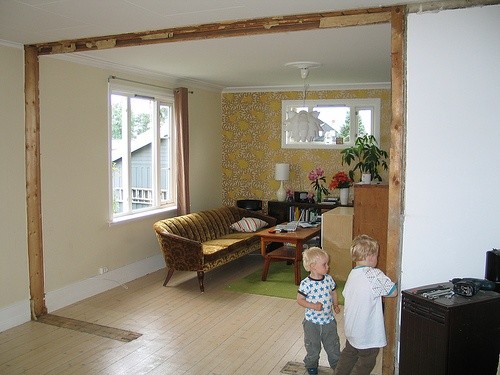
[333,234,397,375]
[297,247,342,375]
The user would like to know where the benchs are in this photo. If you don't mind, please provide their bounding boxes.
[153,206,276,293]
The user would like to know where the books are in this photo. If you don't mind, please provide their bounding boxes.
[317,197,339,206]
[289,205,326,224]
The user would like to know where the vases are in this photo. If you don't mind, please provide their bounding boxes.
[340,188,349,206]
[317,188,321,203]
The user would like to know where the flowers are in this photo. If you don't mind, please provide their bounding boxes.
[308,167,329,195]
[330,171,353,190]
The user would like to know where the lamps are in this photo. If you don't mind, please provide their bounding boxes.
[275,163,289,201]
[283,61,324,143]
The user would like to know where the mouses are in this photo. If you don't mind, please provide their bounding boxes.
[279,229,287,233]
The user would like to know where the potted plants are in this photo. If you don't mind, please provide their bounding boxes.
[340,135,388,184]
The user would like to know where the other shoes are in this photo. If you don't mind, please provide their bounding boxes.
[307,368,319,375]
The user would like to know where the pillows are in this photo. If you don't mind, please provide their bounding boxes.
[230,217,268,232]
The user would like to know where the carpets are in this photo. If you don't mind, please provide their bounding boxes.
[223,261,346,305]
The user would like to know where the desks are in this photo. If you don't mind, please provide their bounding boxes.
[256,221,322,285]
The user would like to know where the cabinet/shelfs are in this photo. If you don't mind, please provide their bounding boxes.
[322,206,354,281]
[399,281,500,375]
[266,200,338,254]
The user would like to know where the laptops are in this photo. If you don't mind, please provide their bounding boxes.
[274,210,304,231]
